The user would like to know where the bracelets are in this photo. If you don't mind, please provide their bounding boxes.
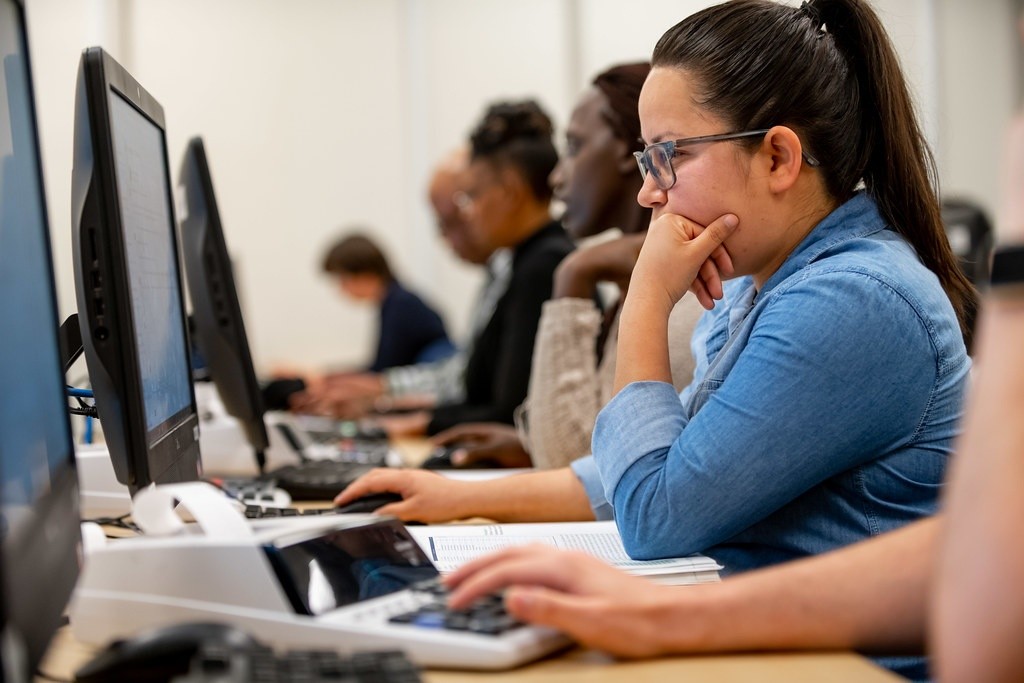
[988,244,1024,286]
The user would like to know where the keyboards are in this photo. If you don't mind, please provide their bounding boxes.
[245,505,335,518]
[252,647,422,683]
[271,459,387,500]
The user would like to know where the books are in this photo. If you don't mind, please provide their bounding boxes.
[405,519,723,586]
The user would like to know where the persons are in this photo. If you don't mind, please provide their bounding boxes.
[327,1,987,681]
[274,61,704,473]
[441,117,1024,683]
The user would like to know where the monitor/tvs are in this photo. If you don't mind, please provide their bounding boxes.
[0,0,271,683]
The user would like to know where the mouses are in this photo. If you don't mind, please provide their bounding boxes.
[421,445,508,469]
[334,491,404,513]
[73,620,288,683]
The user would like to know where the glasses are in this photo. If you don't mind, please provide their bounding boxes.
[633,129,820,190]
